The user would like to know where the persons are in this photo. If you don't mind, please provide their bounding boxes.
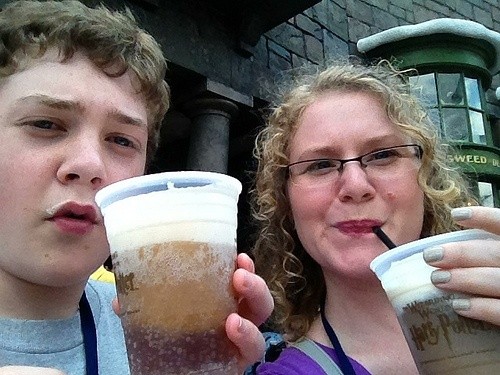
[250,60,500,374]
[0,0,274,375]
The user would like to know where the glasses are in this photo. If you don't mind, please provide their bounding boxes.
[286,142,424,190]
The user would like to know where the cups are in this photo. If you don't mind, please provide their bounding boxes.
[369,228,500,375]
[95,170,243,375]
